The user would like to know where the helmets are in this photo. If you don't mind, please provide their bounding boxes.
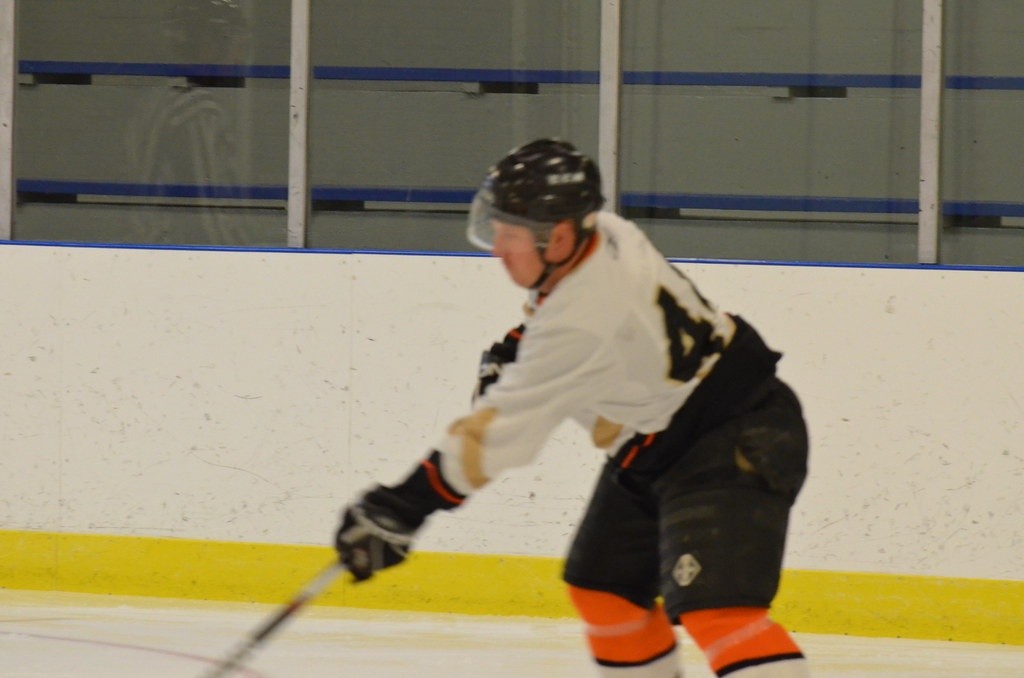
[480,138,608,228]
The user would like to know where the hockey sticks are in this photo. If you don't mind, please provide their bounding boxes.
[206,558,347,676]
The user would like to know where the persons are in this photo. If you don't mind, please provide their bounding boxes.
[334,138,808,678]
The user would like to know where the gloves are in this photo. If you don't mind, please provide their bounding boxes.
[337,485,425,581]
[469,344,514,406]
